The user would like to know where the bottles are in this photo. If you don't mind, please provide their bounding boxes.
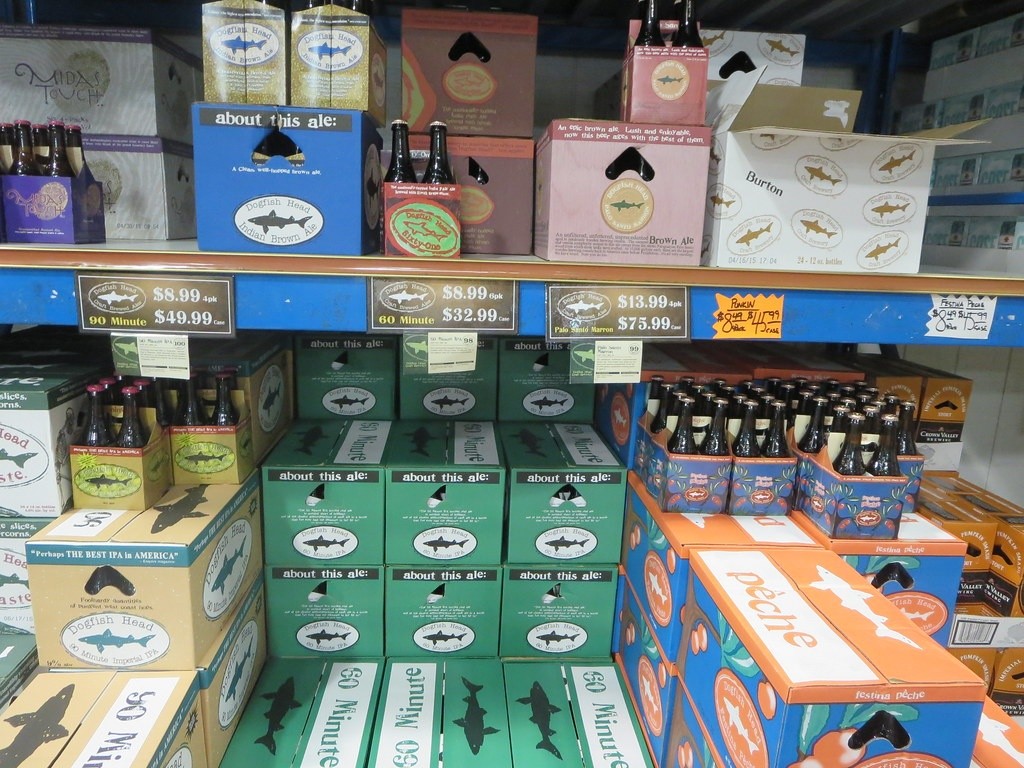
[833,413,866,476]
[115,386,146,448]
[866,414,900,477]
[384,119,418,183]
[97,366,238,427]
[0,120,85,177]
[81,384,113,448]
[421,120,456,184]
[633,0,705,48]
[643,375,917,457]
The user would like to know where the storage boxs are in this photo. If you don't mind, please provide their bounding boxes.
[1,11,1024,768]
